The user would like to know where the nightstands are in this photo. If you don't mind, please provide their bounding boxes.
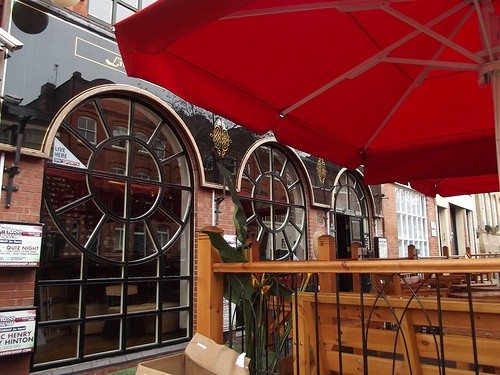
[65,304,109,335]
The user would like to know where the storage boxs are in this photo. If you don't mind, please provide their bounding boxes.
[135,332,251,375]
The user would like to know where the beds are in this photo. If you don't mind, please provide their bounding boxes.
[106,284,180,334]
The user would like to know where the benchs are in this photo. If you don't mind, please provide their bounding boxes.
[291,291,500,375]
